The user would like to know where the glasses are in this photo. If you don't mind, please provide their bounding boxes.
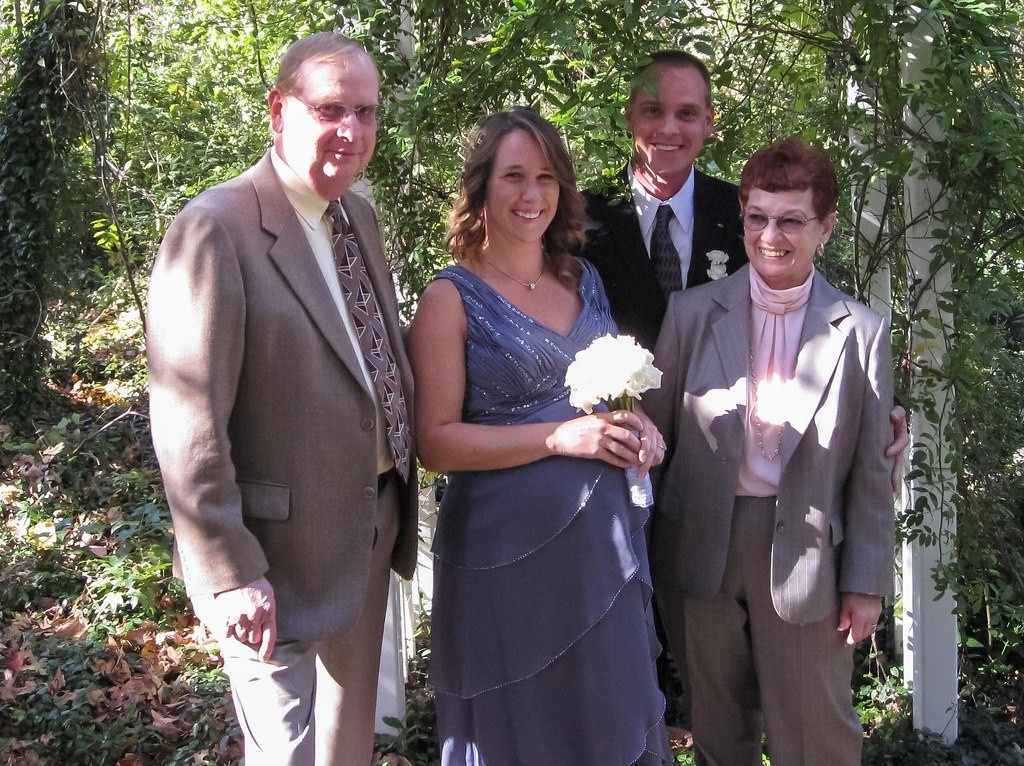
[743,205,824,234]
[285,92,387,127]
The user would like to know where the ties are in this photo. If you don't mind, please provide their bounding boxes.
[325,198,413,482]
[649,205,683,304]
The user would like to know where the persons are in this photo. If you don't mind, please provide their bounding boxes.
[406,108,667,766]
[642,133,895,766]
[577,50,908,733]
[143,34,418,766]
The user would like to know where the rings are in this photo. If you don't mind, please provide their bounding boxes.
[657,445,667,450]
[872,624,877,631]
[257,612,270,627]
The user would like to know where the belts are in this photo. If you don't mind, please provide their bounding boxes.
[377,465,396,497]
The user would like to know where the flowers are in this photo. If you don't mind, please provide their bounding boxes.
[706,250,729,280]
[563,333,664,508]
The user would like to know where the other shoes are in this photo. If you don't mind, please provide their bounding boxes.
[665,725,694,756]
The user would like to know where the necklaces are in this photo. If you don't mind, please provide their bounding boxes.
[481,252,543,289]
[749,336,794,460]
